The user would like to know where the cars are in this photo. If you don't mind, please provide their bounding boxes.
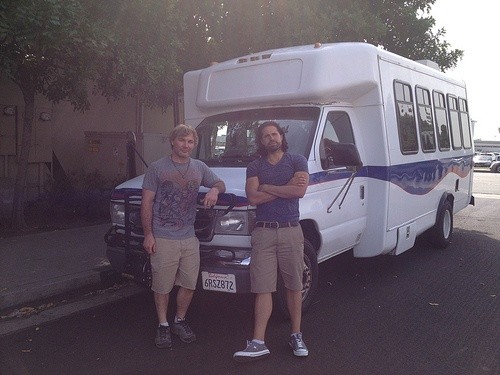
[474,151,500,173]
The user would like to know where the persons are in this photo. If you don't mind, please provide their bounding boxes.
[232,121,309,362]
[140,124,227,348]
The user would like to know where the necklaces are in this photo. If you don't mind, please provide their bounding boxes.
[171,157,191,178]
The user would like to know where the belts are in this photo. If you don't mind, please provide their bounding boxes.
[257,220,298,228]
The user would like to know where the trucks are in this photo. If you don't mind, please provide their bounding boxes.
[104,40,476,324]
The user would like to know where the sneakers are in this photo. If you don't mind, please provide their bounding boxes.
[155,325,172,348]
[289,332,308,356]
[171,316,196,343]
[233,340,270,362]
